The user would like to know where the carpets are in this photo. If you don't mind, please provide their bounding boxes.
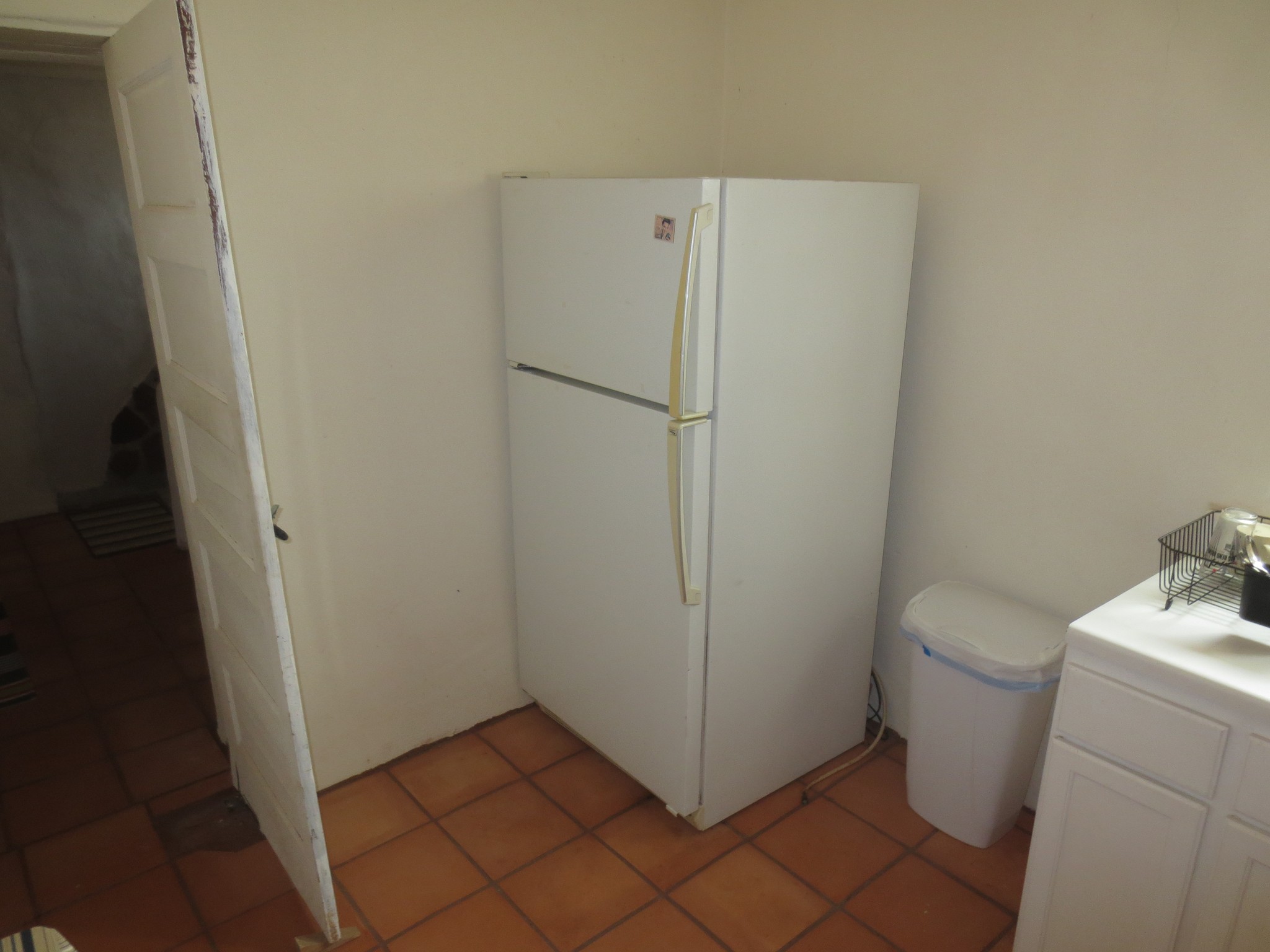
[67,498,178,559]
[0,600,38,708]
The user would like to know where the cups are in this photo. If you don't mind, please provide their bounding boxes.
[1198,507,1258,587]
[1225,522,1270,579]
[1239,563,1270,628]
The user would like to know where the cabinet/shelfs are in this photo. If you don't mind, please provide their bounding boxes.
[1013,646,1270,952]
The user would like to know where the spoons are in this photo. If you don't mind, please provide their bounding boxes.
[1237,547,1262,573]
[1245,535,1270,574]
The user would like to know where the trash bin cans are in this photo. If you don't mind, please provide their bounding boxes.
[900,580,1074,850]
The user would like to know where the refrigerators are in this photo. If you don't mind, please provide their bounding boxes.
[501,176,919,832]
